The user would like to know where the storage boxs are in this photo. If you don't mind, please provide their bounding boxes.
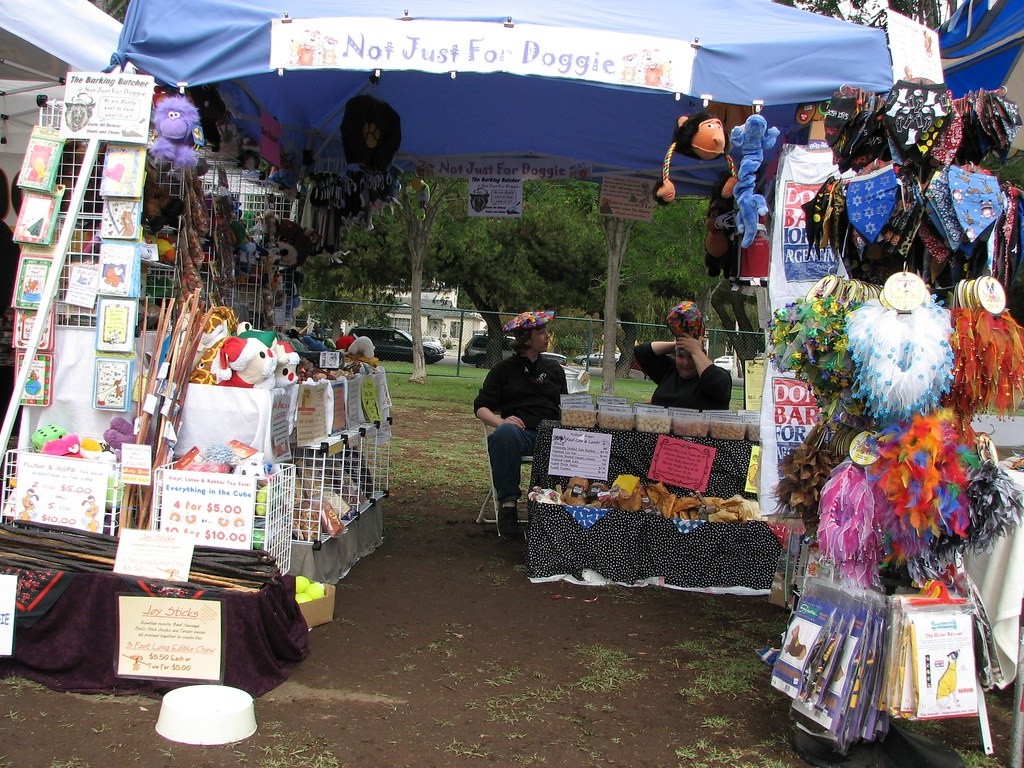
[558,393,760,441]
[294,580,338,628]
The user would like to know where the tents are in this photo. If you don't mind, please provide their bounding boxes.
[0,0,893,473]
[0,0,309,274]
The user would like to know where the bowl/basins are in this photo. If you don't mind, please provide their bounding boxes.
[155,682,258,744]
[559,393,756,444]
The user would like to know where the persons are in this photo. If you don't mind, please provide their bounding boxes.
[633,333,732,410]
[474,310,569,539]
[0,170,27,470]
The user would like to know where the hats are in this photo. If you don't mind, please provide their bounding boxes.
[667,301,706,343]
[502,310,556,338]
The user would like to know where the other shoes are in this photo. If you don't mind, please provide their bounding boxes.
[498,500,519,539]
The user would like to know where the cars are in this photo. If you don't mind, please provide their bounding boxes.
[714,354,744,377]
[574,351,622,368]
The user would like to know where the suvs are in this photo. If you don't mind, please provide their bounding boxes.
[460,333,568,368]
[347,326,445,364]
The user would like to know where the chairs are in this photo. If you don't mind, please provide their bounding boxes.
[477,409,535,538]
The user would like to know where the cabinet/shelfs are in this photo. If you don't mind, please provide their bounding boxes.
[522,417,801,597]
[3,156,392,575]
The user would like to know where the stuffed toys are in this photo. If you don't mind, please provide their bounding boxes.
[149,96,201,168]
[704,199,739,279]
[730,114,780,249]
[336,335,375,359]
[653,112,739,205]
[31,418,137,463]
[189,306,299,390]
